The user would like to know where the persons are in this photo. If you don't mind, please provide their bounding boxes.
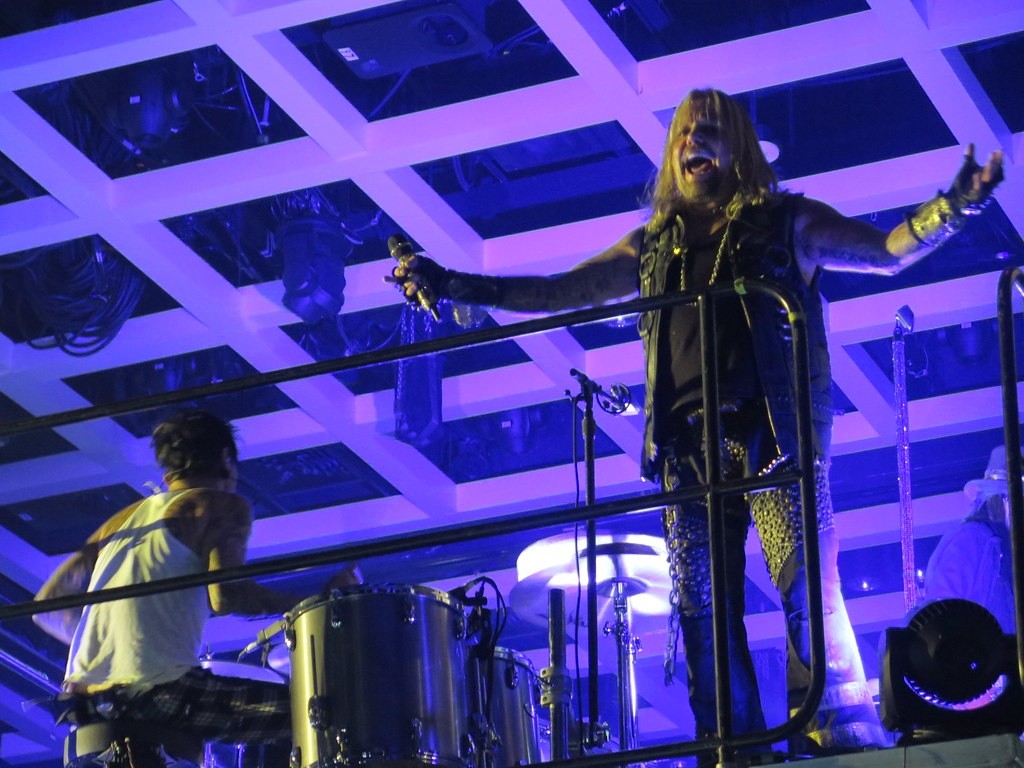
[921,444,1024,633]
[382,81,1007,768]
[33,412,361,768]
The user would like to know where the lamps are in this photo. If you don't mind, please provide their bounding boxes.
[876,595,1024,751]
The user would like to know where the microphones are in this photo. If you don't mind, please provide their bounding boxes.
[608,397,625,411]
[388,233,440,323]
[449,576,483,597]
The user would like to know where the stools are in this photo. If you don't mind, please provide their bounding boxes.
[63,724,206,768]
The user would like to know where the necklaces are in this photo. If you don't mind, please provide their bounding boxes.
[680,214,738,308]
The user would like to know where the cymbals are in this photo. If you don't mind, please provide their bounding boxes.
[199,660,286,685]
[507,552,682,635]
[267,642,291,675]
[516,527,667,583]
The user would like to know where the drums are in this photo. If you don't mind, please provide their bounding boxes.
[202,741,291,768]
[472,646,541,768]
[283,582,471,768]
